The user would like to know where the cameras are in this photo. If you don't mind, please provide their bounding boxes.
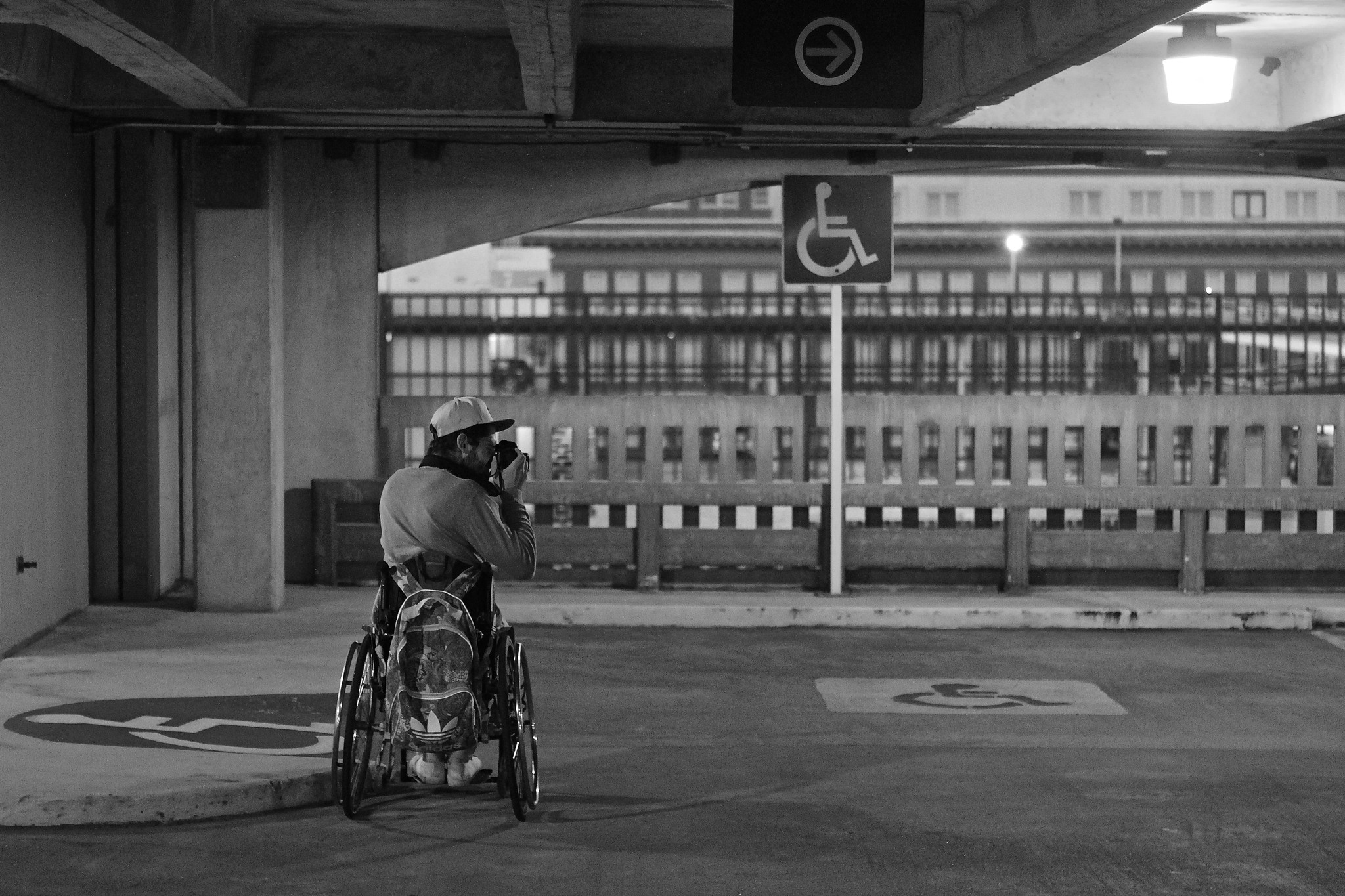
[494,440,530,473]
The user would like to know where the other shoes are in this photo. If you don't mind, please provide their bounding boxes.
[409,754,445,784]
[447,755,483,787]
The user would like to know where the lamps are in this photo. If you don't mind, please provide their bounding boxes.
[1162,19,1238,104]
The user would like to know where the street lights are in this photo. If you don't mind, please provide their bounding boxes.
[1004,229,1024,315]
[1113,217,1123,294]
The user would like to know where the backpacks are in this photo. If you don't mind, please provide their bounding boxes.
[370,563,498,753]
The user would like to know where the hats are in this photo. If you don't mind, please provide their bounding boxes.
[430,396,515,442]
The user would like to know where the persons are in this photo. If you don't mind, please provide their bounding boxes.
[372,396,536,788]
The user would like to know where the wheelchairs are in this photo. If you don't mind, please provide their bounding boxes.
[331,559,539,821]
